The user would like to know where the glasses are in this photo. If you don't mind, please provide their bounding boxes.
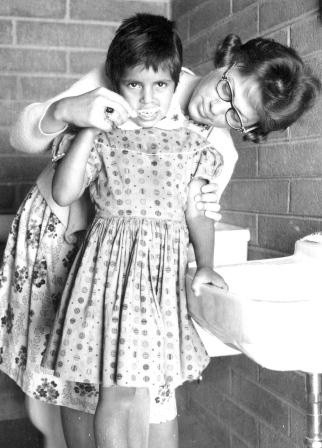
[215,62,259,134]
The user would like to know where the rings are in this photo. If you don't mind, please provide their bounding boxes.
[104,107,114,114]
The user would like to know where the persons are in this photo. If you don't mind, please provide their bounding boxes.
[40,11,230,448]
[1,33,320,448]
